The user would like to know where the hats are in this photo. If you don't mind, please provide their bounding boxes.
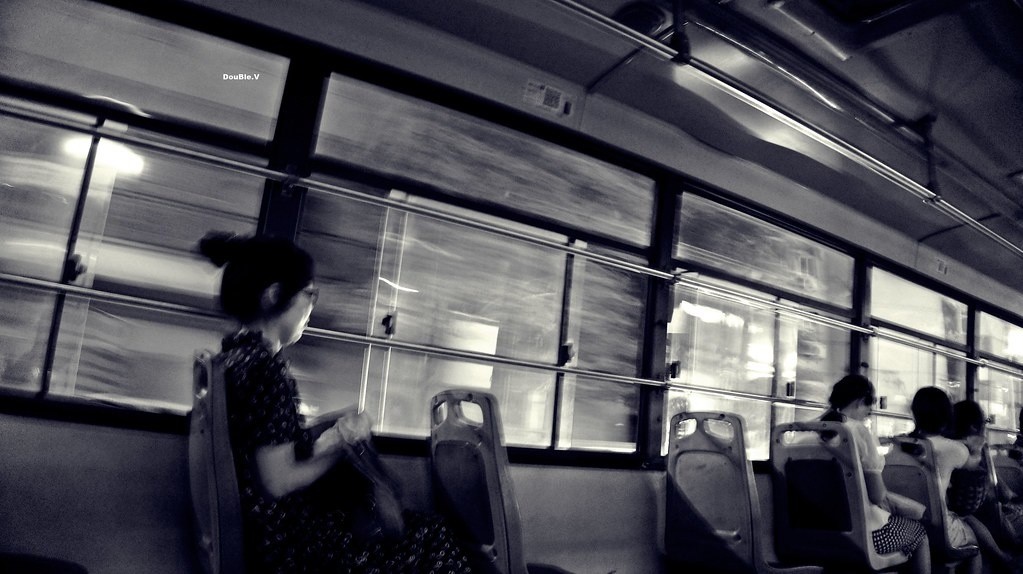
[199,229,314,321]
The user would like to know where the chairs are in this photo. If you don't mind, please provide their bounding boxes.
[877,436,980,574]
[187,347,242,574]
[428,387,574,574]
[989,443,1023,506]
[949,439,1023,557]
[0,551,88,574]
[663,410,823,574]
[770,421,909,574]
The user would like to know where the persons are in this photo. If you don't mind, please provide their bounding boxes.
[195,227,504,574]
[886,385,1023,574]
[790,369,933,574]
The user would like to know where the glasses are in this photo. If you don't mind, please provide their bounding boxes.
[302,288,320,305]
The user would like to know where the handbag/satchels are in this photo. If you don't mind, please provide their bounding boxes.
[887,490,926,518]
[948,469,990,516]
[307,417,404,536]
